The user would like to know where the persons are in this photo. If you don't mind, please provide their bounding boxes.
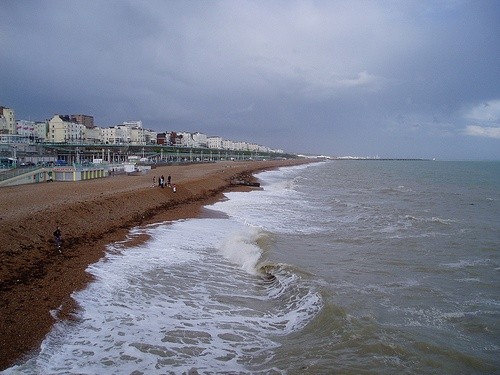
[55,226,62,250]
[153,174,172,189]
[172,184,177,193]
